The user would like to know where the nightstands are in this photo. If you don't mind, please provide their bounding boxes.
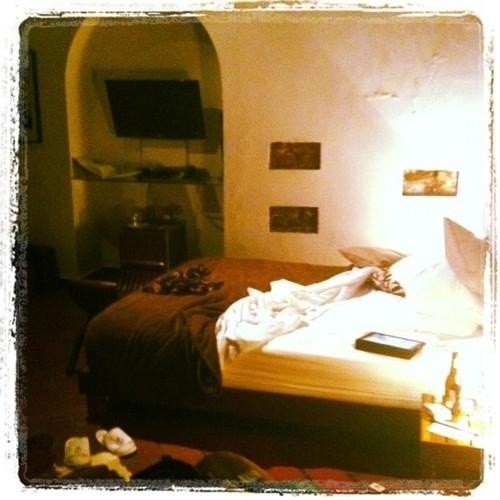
[419,394,483,484]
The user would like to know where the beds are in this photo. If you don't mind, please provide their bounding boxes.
[76,257,483,483]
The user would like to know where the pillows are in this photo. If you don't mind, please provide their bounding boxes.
[338,246,403,268]
[444,218,486,298]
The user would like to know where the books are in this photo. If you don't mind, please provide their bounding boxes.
[73,156,143,180]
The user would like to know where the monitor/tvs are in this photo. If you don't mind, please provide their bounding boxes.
[105,77,207,140]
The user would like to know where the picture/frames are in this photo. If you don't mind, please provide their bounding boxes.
[20,51,42,145]
